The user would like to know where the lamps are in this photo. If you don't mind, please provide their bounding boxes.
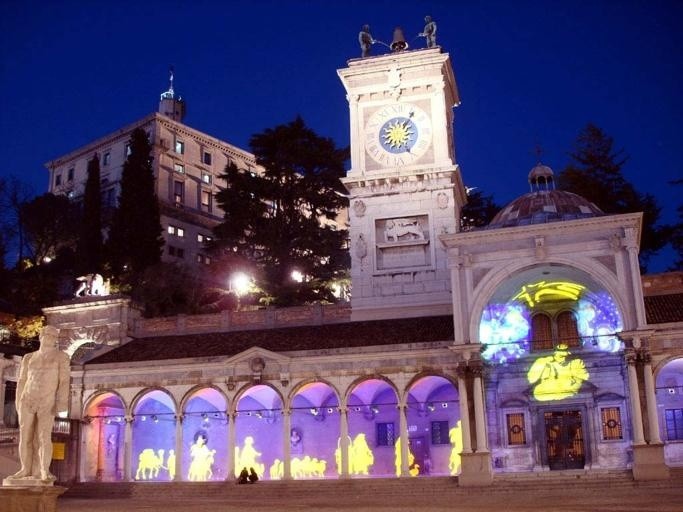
[105,401,448,425]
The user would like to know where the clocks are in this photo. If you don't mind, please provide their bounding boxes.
[363,103,432,167]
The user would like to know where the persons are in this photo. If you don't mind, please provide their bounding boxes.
[526,344,589,400]
[358,24,374,58]
[6,326,70,481]
[423,16,437,48]
